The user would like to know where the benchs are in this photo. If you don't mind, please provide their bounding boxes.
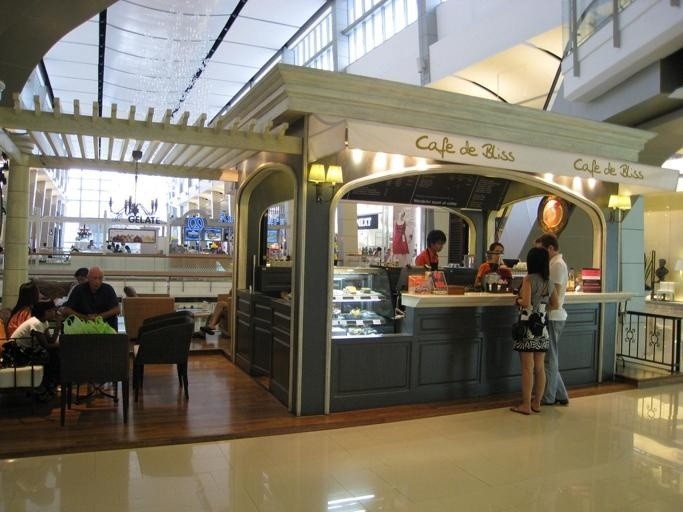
[0,318,50,416]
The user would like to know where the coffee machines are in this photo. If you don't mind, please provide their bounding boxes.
[481,250,504,290]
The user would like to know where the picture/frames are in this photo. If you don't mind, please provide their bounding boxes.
[537,196,569,235]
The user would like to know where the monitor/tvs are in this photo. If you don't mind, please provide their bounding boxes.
[267,230,278,243]
[183,226,203,241]
[204,228,224,241]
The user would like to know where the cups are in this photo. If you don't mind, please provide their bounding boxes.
[449,263,460,270]
[486,280,512,294]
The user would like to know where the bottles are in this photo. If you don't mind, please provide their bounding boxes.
[568,267,581,290]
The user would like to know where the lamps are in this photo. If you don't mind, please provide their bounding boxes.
[107,151,159,216]
[308,163,344,204]
[607,194,633,224]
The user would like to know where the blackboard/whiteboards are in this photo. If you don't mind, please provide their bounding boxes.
[341,173,511,211]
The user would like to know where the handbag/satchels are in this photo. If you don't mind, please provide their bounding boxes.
[511,321,528,340]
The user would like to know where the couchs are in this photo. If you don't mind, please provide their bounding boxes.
[121,292,175,339]
[216,287,231,338]
[27,278,73,322]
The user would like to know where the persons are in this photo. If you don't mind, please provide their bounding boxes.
[474,242,505,289]
[87,240,131,254]
[373,247,381,256]
[656,258,669,301]
[393,210,409,266]
[532,233,569,405]
[384,249,391,261]
[415,230,446,271]
[192,288,232,339]
[510,247,561,414]
[6,265,121,401]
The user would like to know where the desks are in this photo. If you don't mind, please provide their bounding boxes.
[177,302,213,333]
[49,329,133,409]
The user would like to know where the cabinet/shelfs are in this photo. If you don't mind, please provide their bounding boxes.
[331,266,396,336]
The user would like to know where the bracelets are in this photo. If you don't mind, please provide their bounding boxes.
[516,297,522,305]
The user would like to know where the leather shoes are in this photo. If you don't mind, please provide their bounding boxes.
[511,400,568,414]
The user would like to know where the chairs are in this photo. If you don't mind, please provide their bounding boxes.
[137,310,194,387]
[656,281,676,301]
[129,315,194,401]
[59,333,130,426]
